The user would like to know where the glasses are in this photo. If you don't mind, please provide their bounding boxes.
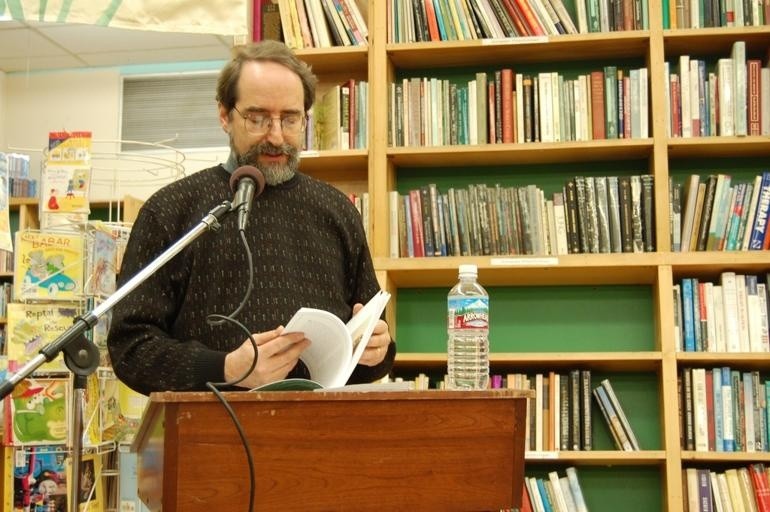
[233,105,308,134]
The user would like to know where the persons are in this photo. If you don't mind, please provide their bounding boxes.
[108,40,398,398]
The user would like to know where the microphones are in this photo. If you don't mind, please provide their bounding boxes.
[229,165,265,231]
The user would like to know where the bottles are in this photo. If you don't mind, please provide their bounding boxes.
[445,265,491,391]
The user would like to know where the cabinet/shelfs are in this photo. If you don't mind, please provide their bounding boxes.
[1,193,148,353]
[251,1,770,511]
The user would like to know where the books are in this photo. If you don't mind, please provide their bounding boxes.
[301,79,368,150]
[349,193,370,241]
[0,126,165,512]
[386,1,650,149]
[676,366,770,451]
[662,1,770,138]
[668,171,770,252]
[390,174,654,259]
[672,272,770,353]
[246,287,394,393]
[682,464,770,511]
[401,369,639,512]
[261,1,369,48]
